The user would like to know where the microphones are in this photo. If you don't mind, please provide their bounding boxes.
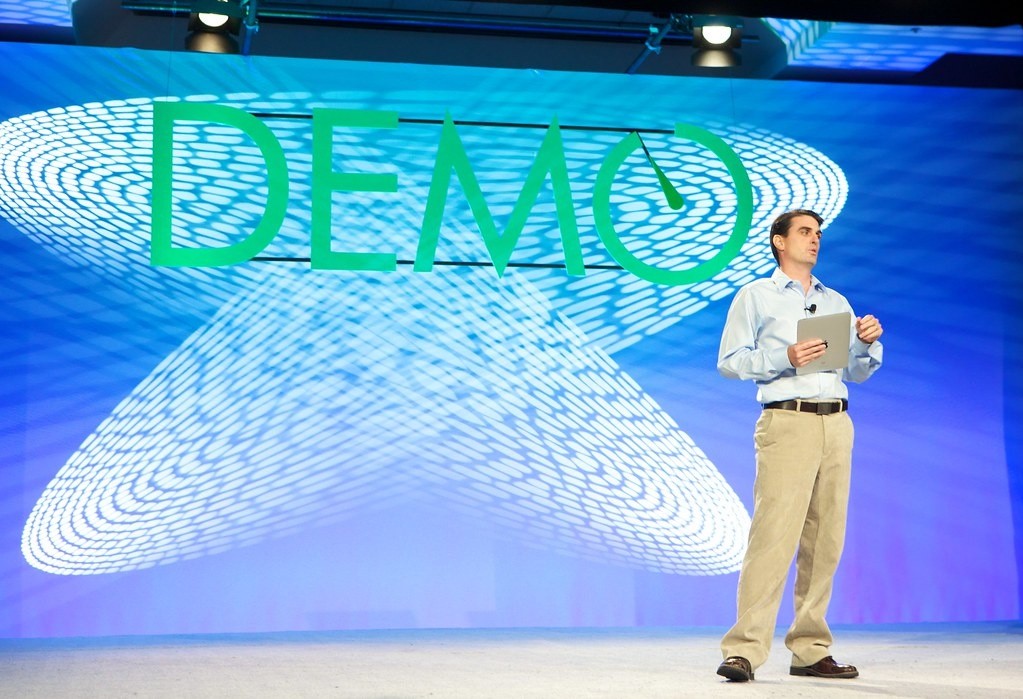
[809,304,816,313]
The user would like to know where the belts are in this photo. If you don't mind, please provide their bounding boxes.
[761,400,848,416]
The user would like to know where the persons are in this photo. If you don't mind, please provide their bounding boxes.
[716,208,886,681]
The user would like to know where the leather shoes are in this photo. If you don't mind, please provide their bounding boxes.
[790,656,859,678]
[717,656,754,682]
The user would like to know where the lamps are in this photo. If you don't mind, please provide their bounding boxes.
[691,49,743,71]
[184,33,241,53]
[693,12,741,48]
[192,0,243,27]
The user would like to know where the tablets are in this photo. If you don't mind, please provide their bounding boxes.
[796,312,851,375]
